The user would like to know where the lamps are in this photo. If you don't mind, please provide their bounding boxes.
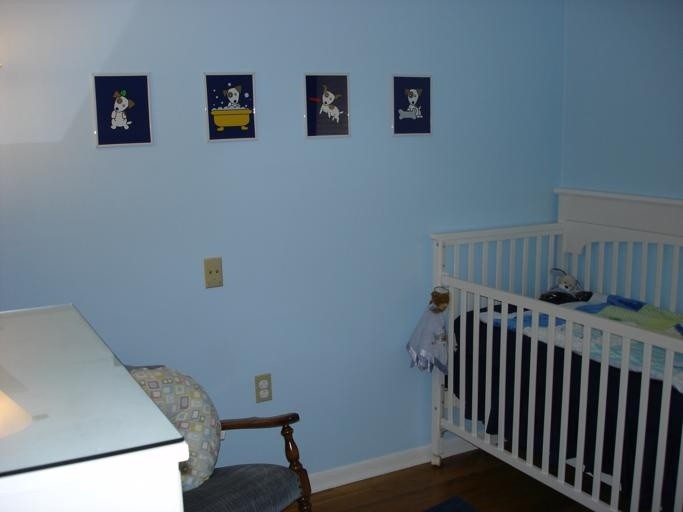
[124,364,312,511]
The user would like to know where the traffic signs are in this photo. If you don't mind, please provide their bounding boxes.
[255,375,272,402]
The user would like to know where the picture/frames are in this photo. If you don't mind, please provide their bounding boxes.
[0,303,191,511]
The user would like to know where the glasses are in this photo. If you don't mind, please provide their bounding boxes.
[0,390,32,439]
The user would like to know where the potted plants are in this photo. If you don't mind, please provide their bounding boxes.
[391,74,433,136]
[202,71,258,143]
[89,71,153,148]
[303,72,351,139]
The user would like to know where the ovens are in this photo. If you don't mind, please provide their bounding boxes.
[430,188,683,512]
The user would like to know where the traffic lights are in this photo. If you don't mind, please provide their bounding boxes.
[130,368,222,492]
